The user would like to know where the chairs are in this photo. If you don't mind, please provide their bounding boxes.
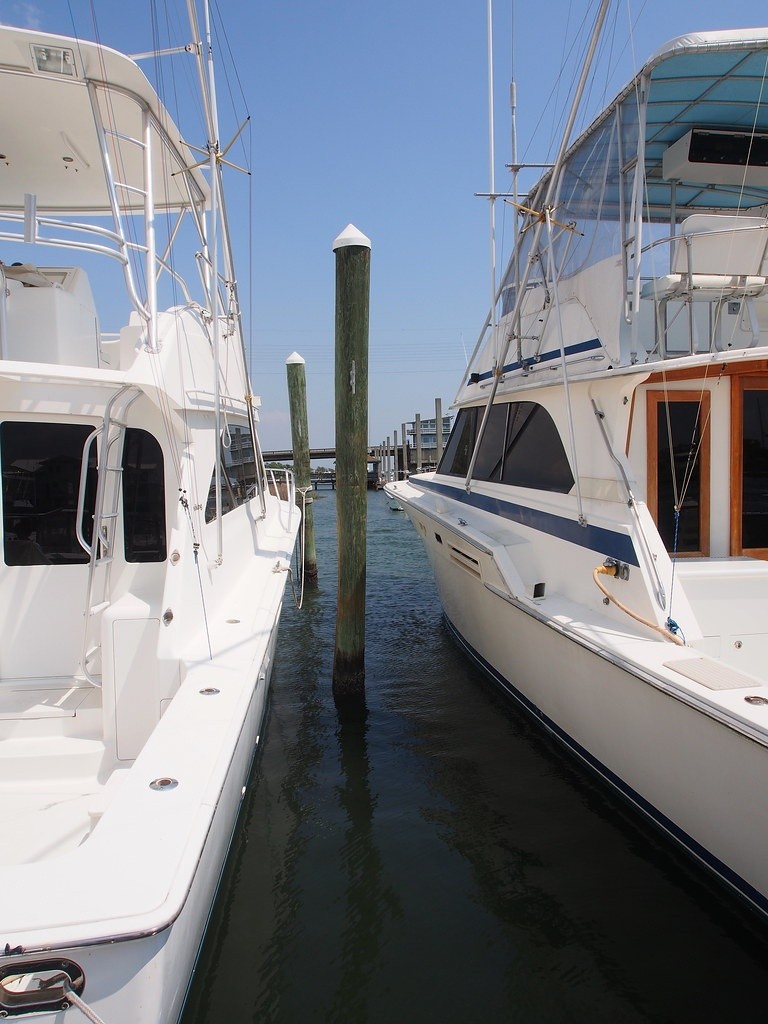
[640,214,768,360]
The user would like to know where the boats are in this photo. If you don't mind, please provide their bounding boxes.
[0,0,305,1024]
[381,0,768,917]
